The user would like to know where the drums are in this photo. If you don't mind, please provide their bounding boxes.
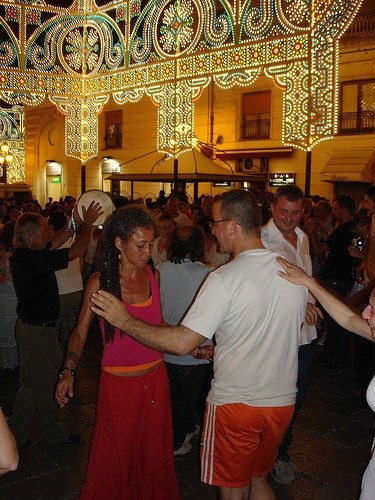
[76,189,116,227]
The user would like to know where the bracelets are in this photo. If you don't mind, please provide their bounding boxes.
[59,367,76,378]
[195,347,202,359]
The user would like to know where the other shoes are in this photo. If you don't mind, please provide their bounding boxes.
[63,432,82,446]
[184,424,200,443]
[174,442,192,456]
[18,438,32,449]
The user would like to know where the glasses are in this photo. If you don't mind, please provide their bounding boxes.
[208,218,241,229]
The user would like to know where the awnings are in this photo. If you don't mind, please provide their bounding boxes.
[321,150,375,183]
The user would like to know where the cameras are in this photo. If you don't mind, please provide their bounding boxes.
[353,237,367,250]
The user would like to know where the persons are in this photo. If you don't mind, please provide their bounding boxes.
[56,206,212,500]
[0,196,83,375]
[254,192,273,225]
[90,189,308,500]
[261,184,324,485]
[8,201,104,447]
[0,407,19,476]
[300,188,375,388]
[84,191,233,456]
[276,256,375,500]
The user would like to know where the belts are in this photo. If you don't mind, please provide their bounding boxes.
[18,315,57,327]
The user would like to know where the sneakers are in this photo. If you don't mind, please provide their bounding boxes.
[270,460,297,486]
